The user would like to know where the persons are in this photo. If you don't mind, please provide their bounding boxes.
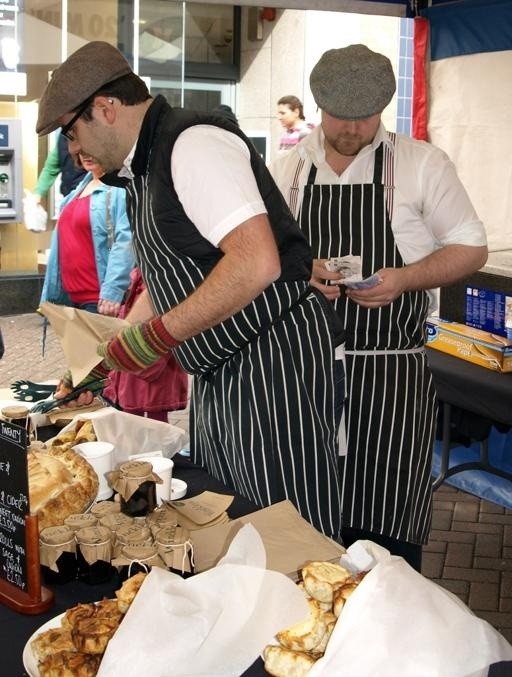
[38,151,141,315]
[34,38,344,546]
[276,93,318,158]
[24,125,87,197]
[264,44,489,574]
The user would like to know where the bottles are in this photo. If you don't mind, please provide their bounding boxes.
[2,406,32,446]
[39,462,191,583]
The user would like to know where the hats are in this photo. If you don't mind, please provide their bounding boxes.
[310,44,397,121]
[36,42,132,137]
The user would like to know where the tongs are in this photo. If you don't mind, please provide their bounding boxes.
[10,377,112,414]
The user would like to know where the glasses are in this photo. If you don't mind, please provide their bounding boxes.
[61,96,117,141]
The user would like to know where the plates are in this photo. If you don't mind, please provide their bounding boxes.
[23,599,118,676]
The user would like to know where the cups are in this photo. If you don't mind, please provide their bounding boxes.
[70,440,116,502]
[134,457,174,506]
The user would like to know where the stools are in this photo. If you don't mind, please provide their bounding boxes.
[424,341,512,492]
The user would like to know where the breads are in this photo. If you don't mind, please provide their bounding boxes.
[31,571,147,677]
[27,417,97,535]
[262,561,374,676]
